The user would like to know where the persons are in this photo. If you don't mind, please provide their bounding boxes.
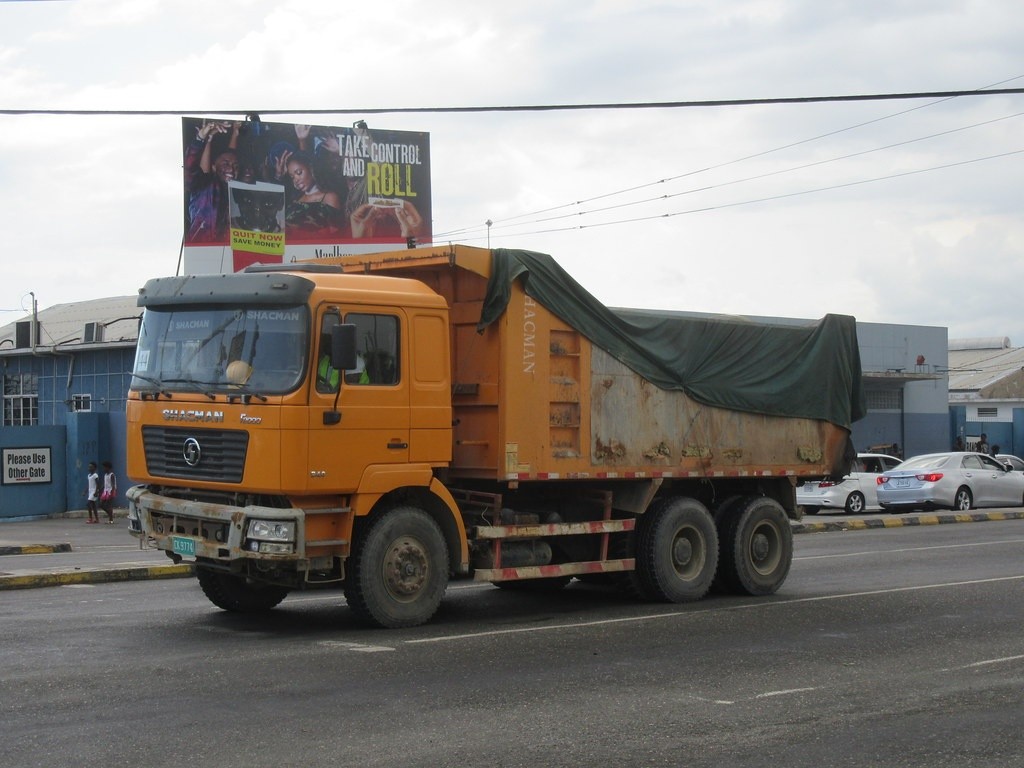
[98,461,117,525]
[183,118,372,244]
[318,341,369,393]
[885,443,903,466]
[82,462,100,524]
[974,433,989,459]
[350,198,423,237]
[951,435,966,452]
[988,445,1000,465]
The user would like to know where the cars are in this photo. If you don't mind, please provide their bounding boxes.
[876,452,1024,512]
[987,454,1024,475]
[795,453,903,516]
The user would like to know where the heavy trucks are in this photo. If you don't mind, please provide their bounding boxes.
[124,243,854,630]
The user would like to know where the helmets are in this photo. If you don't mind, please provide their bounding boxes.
[226,361,253,389]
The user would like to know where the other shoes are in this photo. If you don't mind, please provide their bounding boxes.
[86,517,93,523]
[94,517,99,523]
[108,520,114,524]
